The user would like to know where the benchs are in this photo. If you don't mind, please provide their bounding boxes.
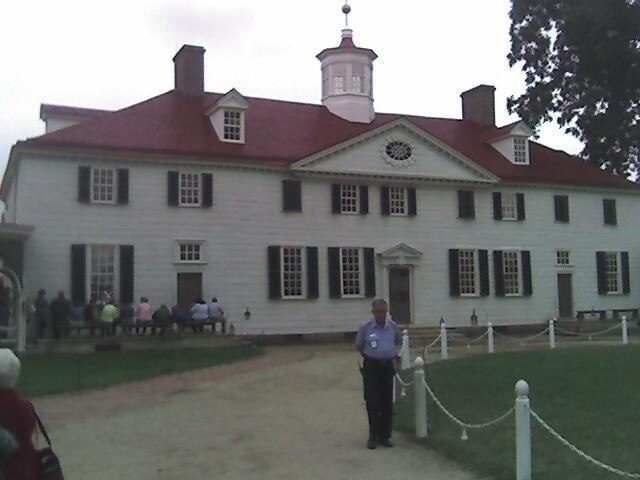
[578,308,639,320]
[55,316,226,339]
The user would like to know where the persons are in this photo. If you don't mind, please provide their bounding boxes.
[35,289,226,339]
[0,348,64,480]
[355,299,403,449]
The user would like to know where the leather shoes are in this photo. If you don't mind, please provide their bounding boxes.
[367,433,393,449]
